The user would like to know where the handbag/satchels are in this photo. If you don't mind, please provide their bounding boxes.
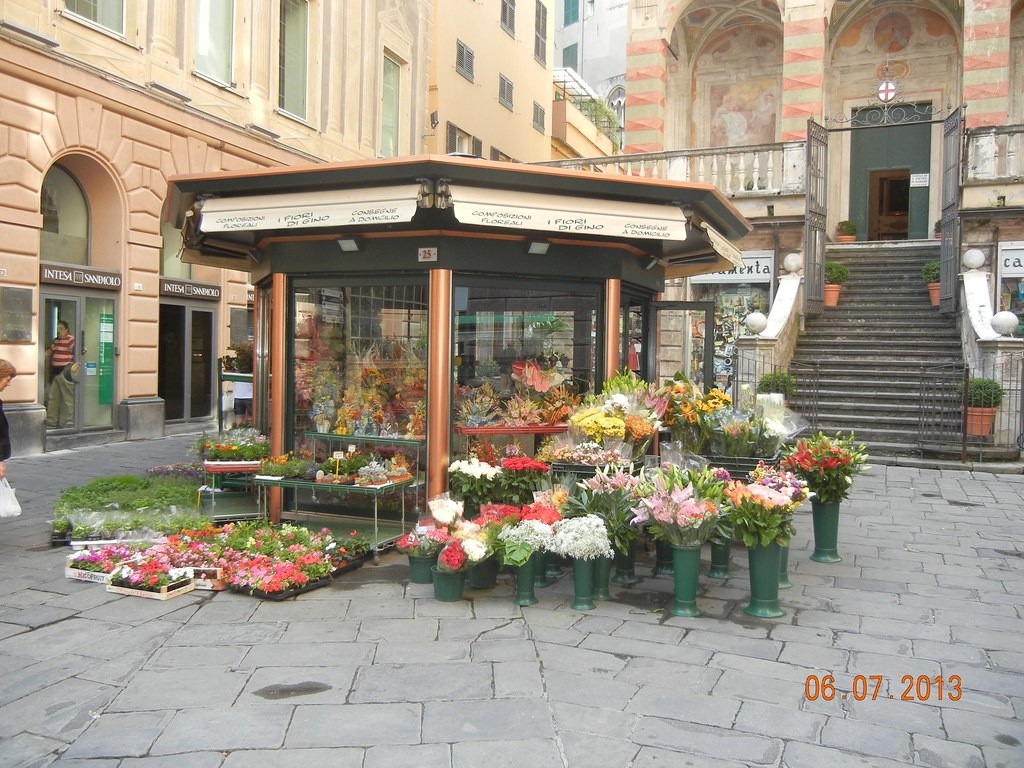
[0,477,22,518]
[53,366,64,375]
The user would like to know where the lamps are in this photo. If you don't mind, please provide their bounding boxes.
[430,110,439,129]
[525,239,552,256]
[336,236,360,255]
[642,257,659,272]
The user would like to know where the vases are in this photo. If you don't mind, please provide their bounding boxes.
[467,560,497,589]
[430,565,464,602]
[741,540,785,614]
[612,539,640,583]
[672,549,699,616]
[704,534,732,579]
[593,544,613,601]
[779,541,794,588]
[808,505,842,563]
[514,552,539,605]
[545,549,563,576]
[570,555,596,611]
[534,551,549,588]
[408,555,436,584]
[651,540,674,575]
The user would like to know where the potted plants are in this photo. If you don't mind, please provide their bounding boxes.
[933,219,941,239]
[823,262,848,307]
[223,344,253,374]
[258,448,412,494]
[311,336,427,440]
[195,426,271,464]
[956,378,1005,436]
[51,473,209,543]
[834,221,857,242]
[922,260,941,306]
[757,372,796,406]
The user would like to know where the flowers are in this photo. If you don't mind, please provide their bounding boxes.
[724,479,804,537]
[629,480,732,547]
[446,364,772,482]
[548,513,615,560]
[745,460,817,515]
[472,504,521,525]
[462,532,493,561]
[498,519,552,554]
[437,538,465,570]
[575,462,640,540]
[396,527,450,555]
[780,430,872,505]
[521,503,562,525]
[427,498,463,524]
[65,525,374,601]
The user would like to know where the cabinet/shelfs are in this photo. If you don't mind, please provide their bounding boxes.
[253,478,416,565]
[201,458,267,524]
[305,431,426,513]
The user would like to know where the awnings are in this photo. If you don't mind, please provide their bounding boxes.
[435,182,744,281]
[177,180,433,274]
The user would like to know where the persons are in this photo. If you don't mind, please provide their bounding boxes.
[45,320,76,428]
[0,358,17,481]
[233,381,254,427]
[500,340,519,391]
